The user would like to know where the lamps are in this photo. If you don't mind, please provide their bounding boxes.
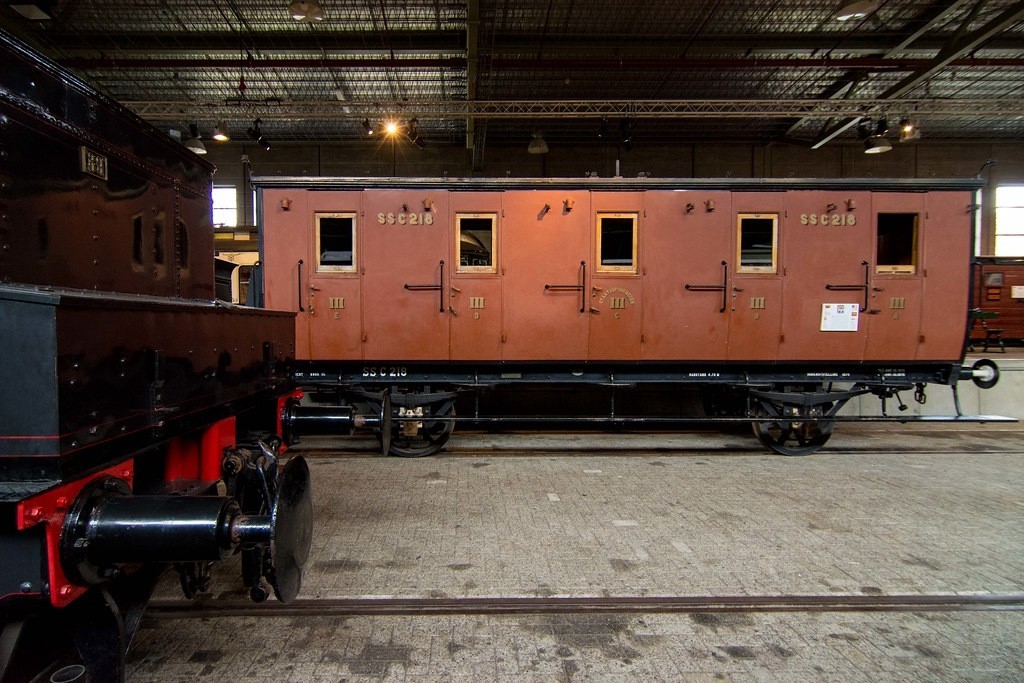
[406,119,426,151]
[246,117,271,150]
[620,118,632,142]
[363,117,374,135]
[185,122,206,155]
[857,118,892,154]
[899,116,915,132]
[598,116,608,137]
[527,129,548,154]
[213,120,230,143]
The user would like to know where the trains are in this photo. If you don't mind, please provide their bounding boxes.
[0,29,315,683]
[214,172,1020,457]
